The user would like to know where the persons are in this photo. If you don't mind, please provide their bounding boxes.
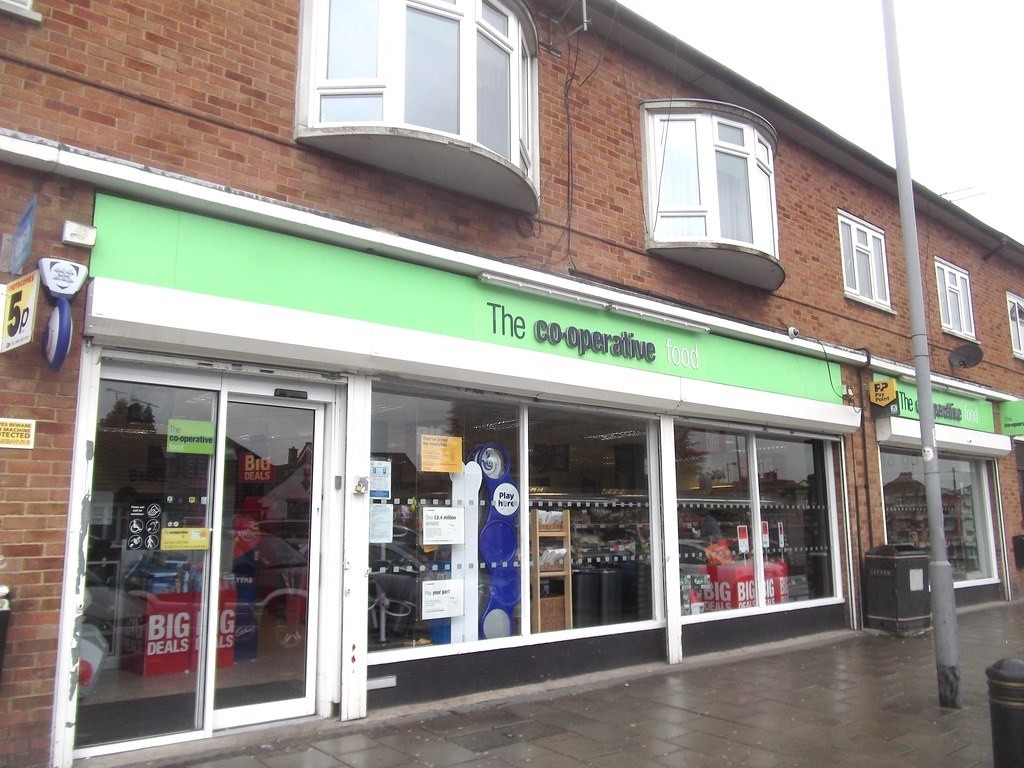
[692,508,732,563]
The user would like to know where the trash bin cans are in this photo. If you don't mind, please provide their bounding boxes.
[1013,535,1024,568]
[863,543,932,631]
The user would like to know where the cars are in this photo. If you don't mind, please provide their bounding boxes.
[60,511,818,678]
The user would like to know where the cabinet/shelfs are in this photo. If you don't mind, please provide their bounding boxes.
[78,537,128,670]
[527,509,573,634]
